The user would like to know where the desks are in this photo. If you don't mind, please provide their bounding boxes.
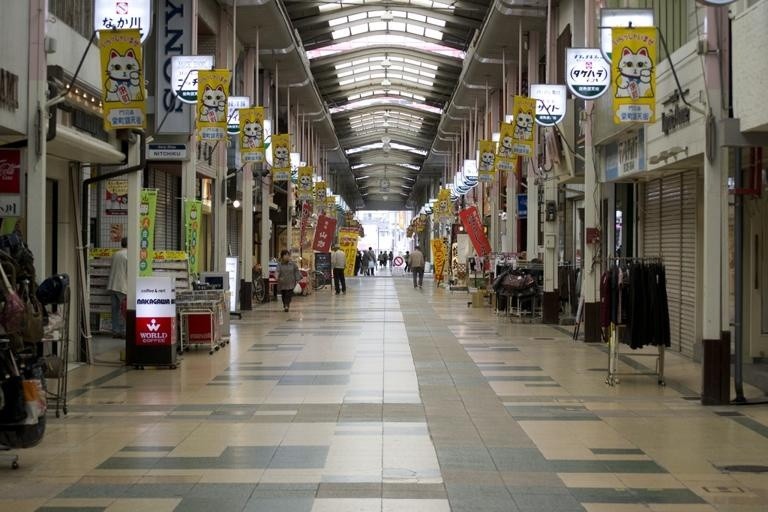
[493,289,544,321]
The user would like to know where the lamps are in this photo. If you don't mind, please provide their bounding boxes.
[648,147,688,166]
[226,197,240,208]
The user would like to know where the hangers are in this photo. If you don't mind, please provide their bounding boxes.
[603,257,663,269]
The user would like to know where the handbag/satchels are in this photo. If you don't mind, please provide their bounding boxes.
[489,266,538,297]
[0,231,64,443]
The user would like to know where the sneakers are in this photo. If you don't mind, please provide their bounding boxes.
[284,306,289,312]
[413,285,423,288]
[354,272,374,276]
[335,291,346,295]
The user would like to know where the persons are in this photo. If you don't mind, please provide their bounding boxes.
[404,251,411,272]
[273,249,302,311]
[407,246,425,289]
[354,247,393,276]
[331,244,346,295]
[106,237,128,340]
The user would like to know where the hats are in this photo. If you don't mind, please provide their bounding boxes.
[332,243,341,249]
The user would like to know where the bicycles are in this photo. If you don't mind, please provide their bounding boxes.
[251,265,267,303]
[311,270,326,291]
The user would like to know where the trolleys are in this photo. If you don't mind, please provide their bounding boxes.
[175,289,230,355]
[43,286,71,418]
